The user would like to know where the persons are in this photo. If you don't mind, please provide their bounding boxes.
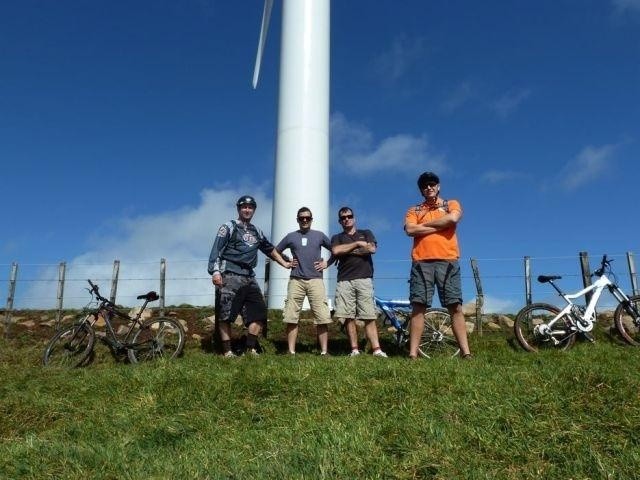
[404,171,474,360]
[208,196,294,362]
[330,207,389,358]
[275,207,336,356]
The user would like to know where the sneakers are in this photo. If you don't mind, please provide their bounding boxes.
[351,351,388,359]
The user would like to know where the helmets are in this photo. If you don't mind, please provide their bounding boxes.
[237,195,256,206]
[418,172,440,185]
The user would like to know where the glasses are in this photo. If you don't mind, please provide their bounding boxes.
[340,214,353,220]
[298,216,312,222]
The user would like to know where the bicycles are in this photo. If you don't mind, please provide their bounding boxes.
[43,281,186,371]
[316,278,461,362]
[514,253,640,355]
